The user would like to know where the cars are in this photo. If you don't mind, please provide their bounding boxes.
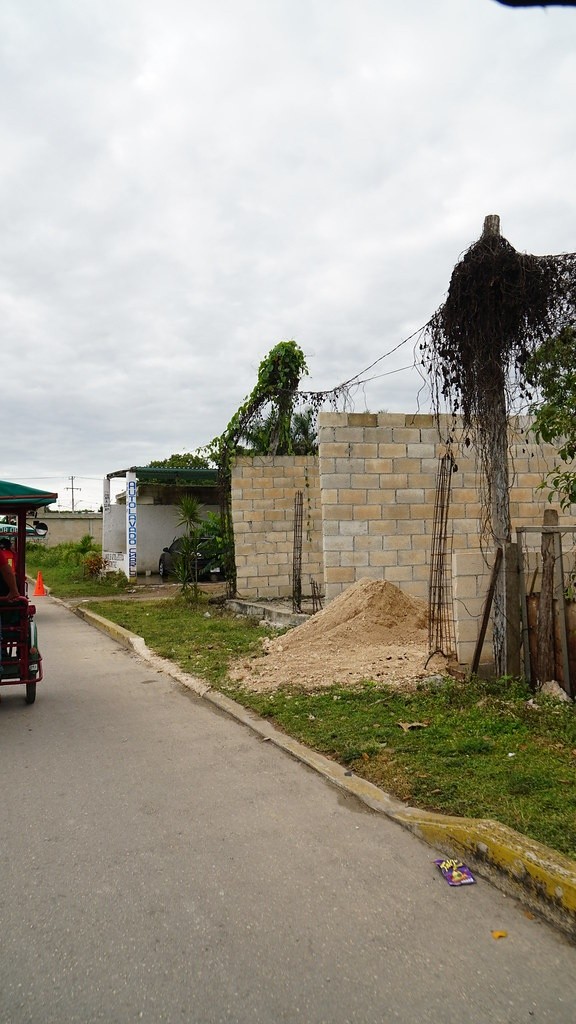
[158,535,224,582]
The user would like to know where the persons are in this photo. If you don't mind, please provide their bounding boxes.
[0,537,22,603]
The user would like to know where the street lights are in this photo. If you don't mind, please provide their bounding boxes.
[58,500,83,514]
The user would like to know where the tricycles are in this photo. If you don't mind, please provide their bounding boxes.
[0,480,58,703]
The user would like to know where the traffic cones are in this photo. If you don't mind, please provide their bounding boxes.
[32,569,47,597]
